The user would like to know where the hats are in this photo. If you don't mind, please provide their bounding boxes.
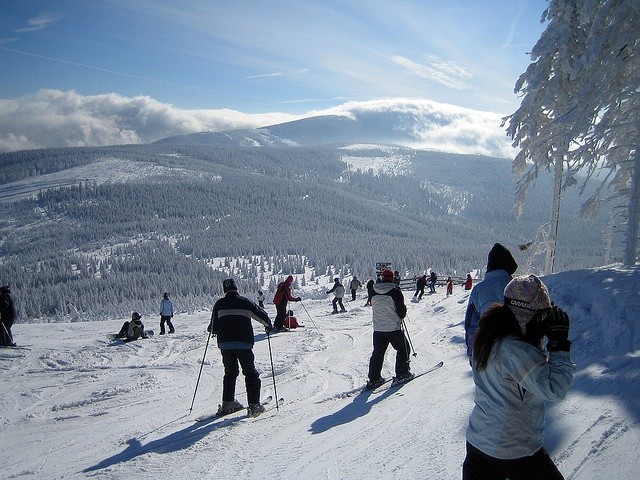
[487,243,518,274]
[0,286,9,293]
[504,274,551,333]
[382,270,393,282]
[334,278,339,281]
[288,275,293,282]
[132,312,142,319]
[223,279,237,293]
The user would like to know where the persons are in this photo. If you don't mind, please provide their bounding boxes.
[465,243,518,367]
[257,290,266,309]
[273,275,302,331]
[427,269,437,293]
[116,312,149,342]
[0,286,16,346]
[364,277,374,307]
[411,275,428,300]
[159,291,175,336]
[462,274,472,290]
[393,271,401,286]
[326,278,345,314]
[446,276,453,295]
[207,278,272,413]
[376,273,382,284]
[285,310,298,329]
[462,274,576,480]
[367,269,412,385]
[349,276,362,300]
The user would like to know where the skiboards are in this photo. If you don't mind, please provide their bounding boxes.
[347,361,444,394]
[195,396,284,423]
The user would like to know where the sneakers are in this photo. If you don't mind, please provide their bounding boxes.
[371,377,385,384]
[340,309,345,311]
[168,330,174,333]
[248,405,265,413]
[332,311,338,313]
[160,332,165,335]
[223,401,240,412]
[397,372,411,378]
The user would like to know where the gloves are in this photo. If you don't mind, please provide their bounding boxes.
[326,291,330,294]
[297,297,301,301]
[545,306,571,352]
[267,325,272,332]
